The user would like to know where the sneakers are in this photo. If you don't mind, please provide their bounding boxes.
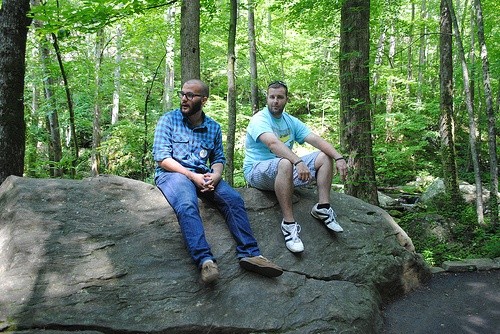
[239,254,284,277]
[281,218,306,253]
[201,262,220,284]
[311,203,345,233]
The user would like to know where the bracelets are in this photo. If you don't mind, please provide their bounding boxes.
[294,160,303,166]
[335,157,344,161]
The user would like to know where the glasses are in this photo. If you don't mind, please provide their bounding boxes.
[267,80,288,89]
[177,91,202,100]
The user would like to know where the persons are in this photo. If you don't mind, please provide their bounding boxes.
[243,81,347,253]
[153,79,284,284]
